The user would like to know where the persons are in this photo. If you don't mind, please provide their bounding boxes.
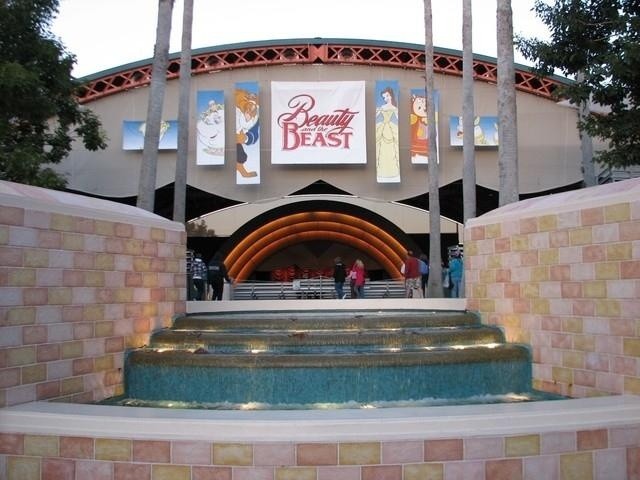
[189,252,234,301]
[400,250,463,298]
[331,256,348,299]
[347,259,366,299]
